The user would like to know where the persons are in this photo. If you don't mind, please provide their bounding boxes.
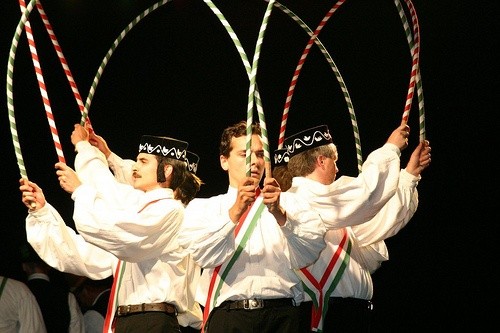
[78,279,111,333]
[24,249,85,333]
[19,123,204,333]
[272,124,431,332]
[179,121,326,333]
[0,277,47,333]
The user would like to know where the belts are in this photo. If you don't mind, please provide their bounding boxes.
[115,303,176,315]
[216,298,293,310]
[303,303,373,312]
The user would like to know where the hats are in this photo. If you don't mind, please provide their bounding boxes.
[283,125,333,160]
[273,148,290,168]
[138,135,187,162]
[185,151,199,175]
